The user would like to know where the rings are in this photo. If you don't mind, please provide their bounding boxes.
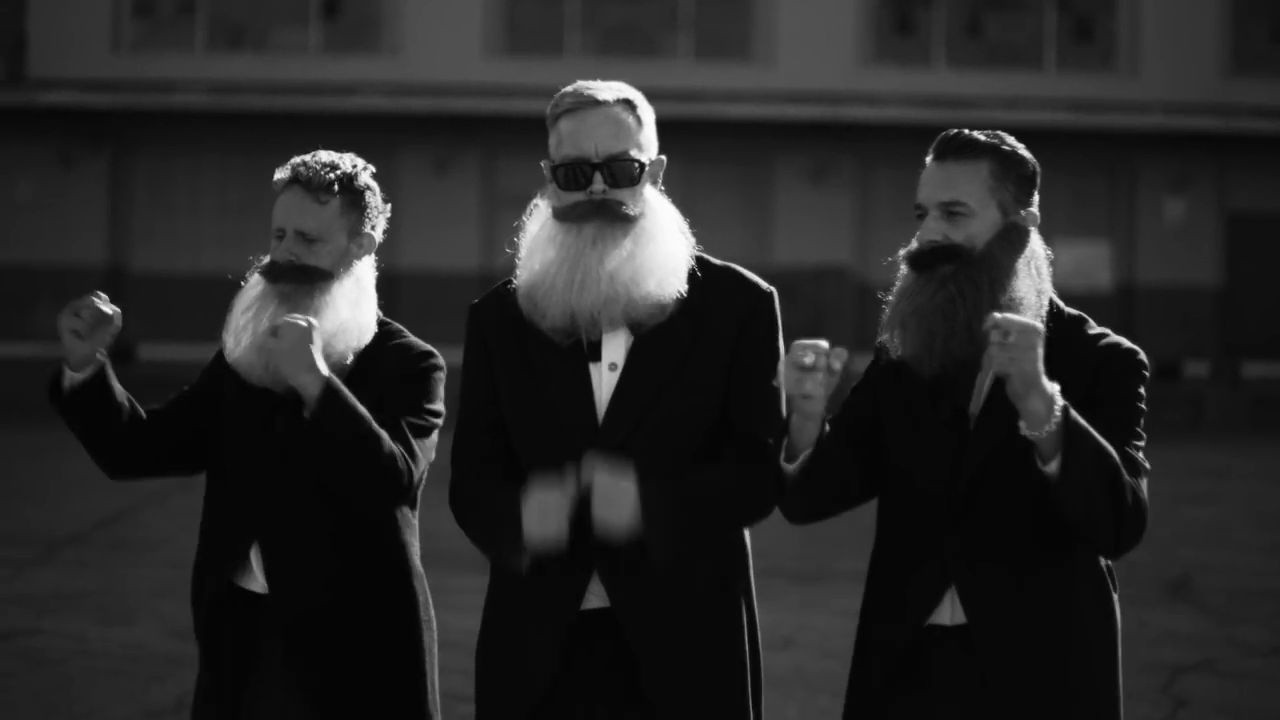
[801,352,816,368]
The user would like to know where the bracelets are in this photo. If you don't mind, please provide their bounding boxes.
[1020,382,1064,439]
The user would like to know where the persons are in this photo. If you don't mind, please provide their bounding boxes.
[778,131,1150,719]
[59,150,446,719]
[446,79,786,718]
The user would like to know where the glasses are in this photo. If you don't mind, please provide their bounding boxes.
[551,156,655,193]
